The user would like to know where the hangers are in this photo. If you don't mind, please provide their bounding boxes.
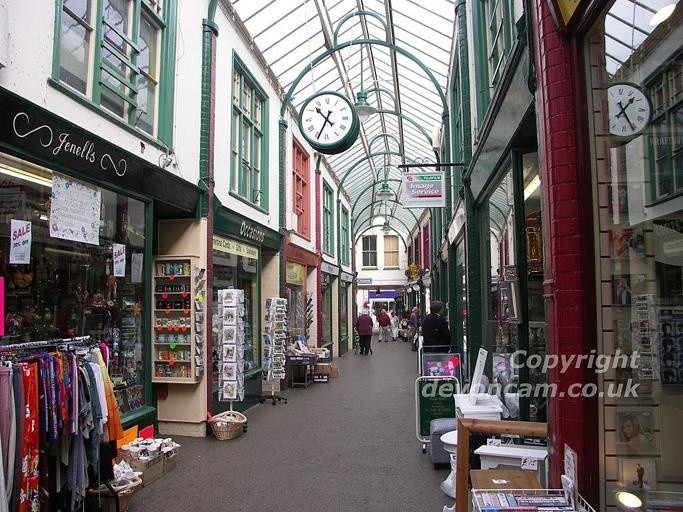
[0,337,99,367]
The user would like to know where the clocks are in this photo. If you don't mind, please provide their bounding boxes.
[607,82,653,148]
[298,91,360,155]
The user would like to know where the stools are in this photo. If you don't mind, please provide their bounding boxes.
[430,417,457,470]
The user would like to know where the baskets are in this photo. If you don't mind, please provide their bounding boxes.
[208,411,247,440]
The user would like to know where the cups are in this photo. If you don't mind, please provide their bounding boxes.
[120,437,173,459]
[154,317,191,378]
[155,283,190,310]
[156,262,190,276]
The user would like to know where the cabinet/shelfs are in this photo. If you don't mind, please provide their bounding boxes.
[152,255,201,385]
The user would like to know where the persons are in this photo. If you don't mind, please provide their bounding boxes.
[354,299,450,355]
[222,289,237,400]
[608,155,683,490]
[236,374,245,389]
[260,298,272,380]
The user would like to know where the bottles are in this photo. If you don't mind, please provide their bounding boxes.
[185,278,189,292]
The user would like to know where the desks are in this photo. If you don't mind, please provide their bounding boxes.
[288,355,316,389]
[470,469,546,492]
[454,393,504,422]
[473,445,549,493]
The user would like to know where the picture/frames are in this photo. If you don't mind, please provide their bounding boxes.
[456,417,548,512]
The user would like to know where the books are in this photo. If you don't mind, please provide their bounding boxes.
[560,473,576,511]
[472,491,587,512]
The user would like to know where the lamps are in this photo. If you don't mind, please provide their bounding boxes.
[377,154,394,203]
[353,43,377,122]
[380,201,391,234]
